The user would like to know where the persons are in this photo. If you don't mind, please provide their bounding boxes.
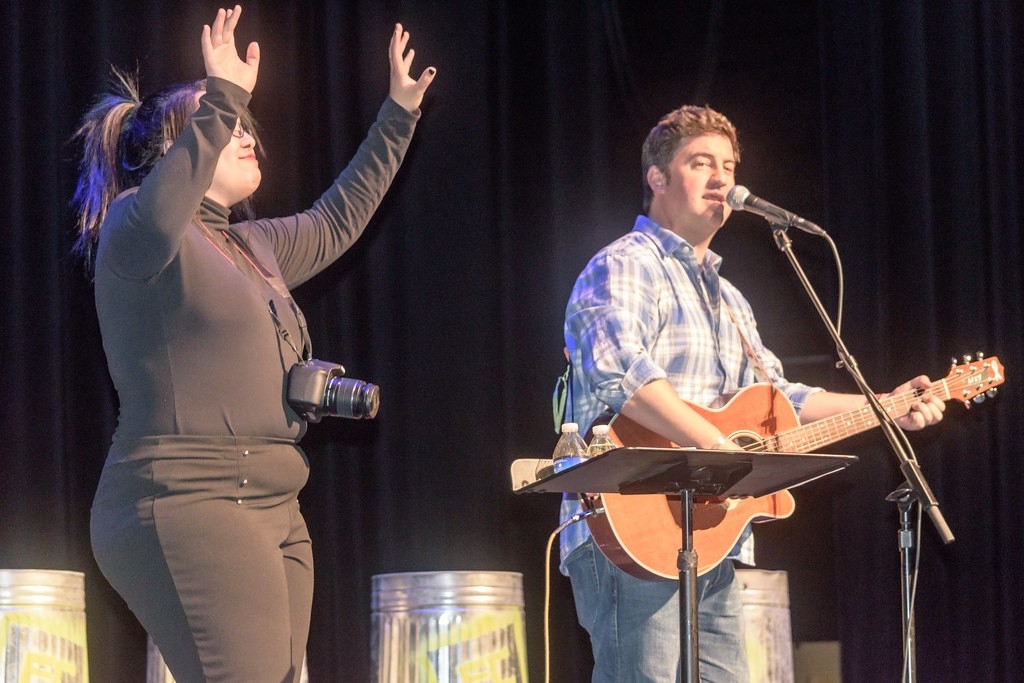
[73,3,438,683]
[558,102,945,683]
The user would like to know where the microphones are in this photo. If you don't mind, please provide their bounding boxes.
[726,185,826,235]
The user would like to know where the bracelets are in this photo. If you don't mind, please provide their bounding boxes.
[710,433,726,450]
[865,393,881,405]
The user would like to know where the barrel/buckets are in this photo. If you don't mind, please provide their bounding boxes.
[369,571,528,683]
[733,568,795,683]
[0,568,89,683]
[146,633,308,683]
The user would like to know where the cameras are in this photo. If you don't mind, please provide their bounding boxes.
[287,359,380,423]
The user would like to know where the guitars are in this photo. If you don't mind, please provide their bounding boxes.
[577,349,1005,581]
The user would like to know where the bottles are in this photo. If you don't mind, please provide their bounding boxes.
[587,425,617,461]
[551,423,589,474]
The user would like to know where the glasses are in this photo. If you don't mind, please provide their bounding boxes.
[232,123,254,138]
